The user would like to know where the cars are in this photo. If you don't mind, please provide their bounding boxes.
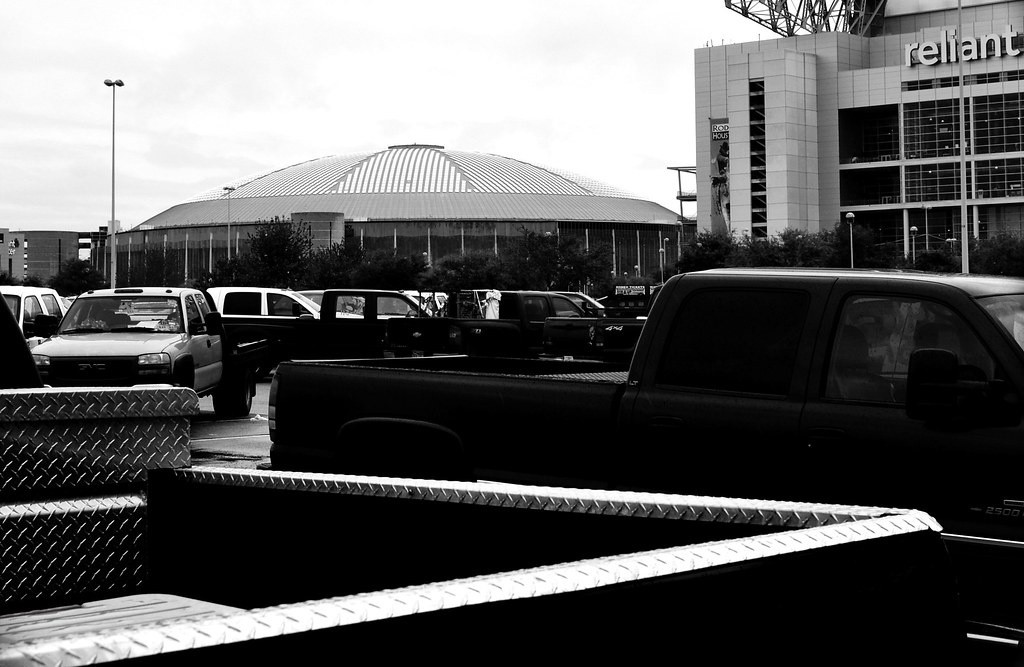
[0,284,605,377]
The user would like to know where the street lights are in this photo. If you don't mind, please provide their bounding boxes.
[223,187,236,257]
[104,79,124,288]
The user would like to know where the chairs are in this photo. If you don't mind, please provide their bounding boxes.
[835,326,896,403]
[97,311,179,329]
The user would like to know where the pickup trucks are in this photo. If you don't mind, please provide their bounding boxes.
[32,288,278,419]
[264,265,1023,657]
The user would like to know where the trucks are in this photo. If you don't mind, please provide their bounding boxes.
[0,294,962,666]
